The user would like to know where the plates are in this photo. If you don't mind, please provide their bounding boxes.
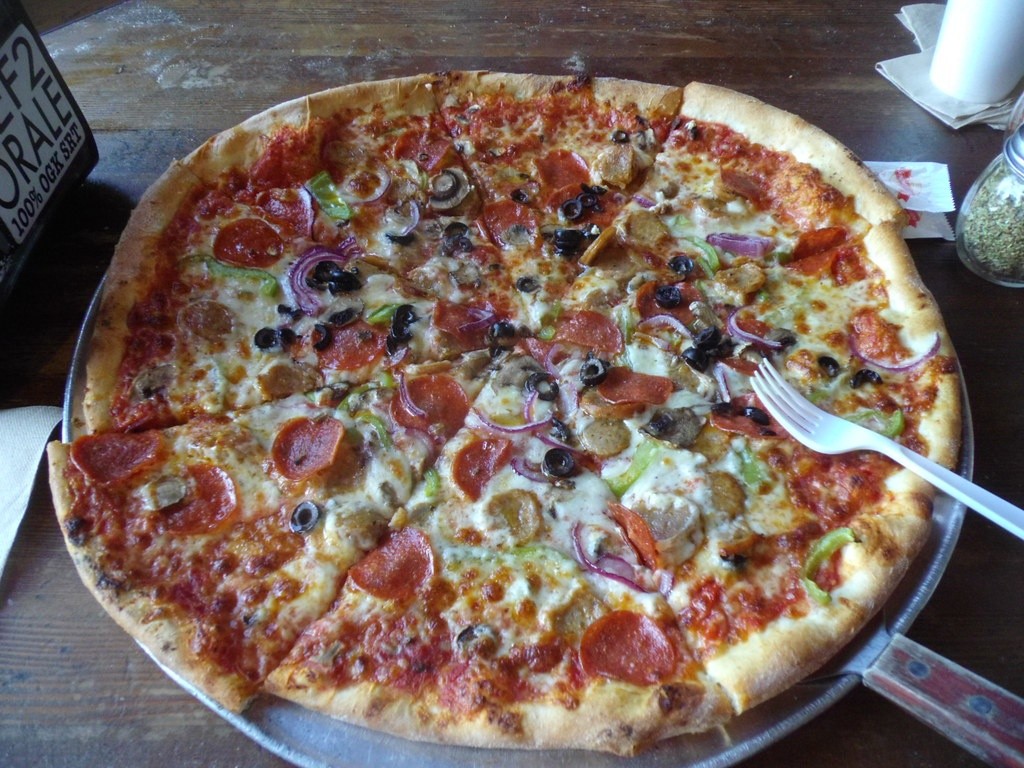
[62,256,1024,768]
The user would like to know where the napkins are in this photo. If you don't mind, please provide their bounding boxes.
[876,4,1024,132]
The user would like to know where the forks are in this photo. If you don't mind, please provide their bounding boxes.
[749,357,1024,540]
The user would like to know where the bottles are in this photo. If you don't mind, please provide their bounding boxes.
[956,124,1024,289]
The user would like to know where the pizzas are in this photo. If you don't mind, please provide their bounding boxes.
[48,68,963,756]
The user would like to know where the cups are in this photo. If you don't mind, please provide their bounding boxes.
[930,0,1024,107]
